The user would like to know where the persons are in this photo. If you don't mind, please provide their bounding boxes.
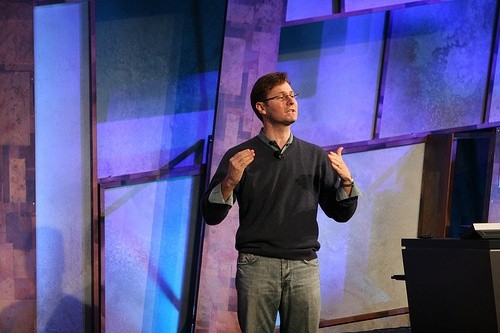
[201,72,360,333]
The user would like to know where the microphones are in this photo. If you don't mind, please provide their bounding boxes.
[276,152,283,159]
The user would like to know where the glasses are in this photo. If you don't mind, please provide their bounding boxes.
[261,93,300,103]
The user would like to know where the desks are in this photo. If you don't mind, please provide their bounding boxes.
[392,238,500,333]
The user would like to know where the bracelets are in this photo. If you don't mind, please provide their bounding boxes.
[341,179,354,188]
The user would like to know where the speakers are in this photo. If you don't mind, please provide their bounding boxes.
[418,132,496,238]
